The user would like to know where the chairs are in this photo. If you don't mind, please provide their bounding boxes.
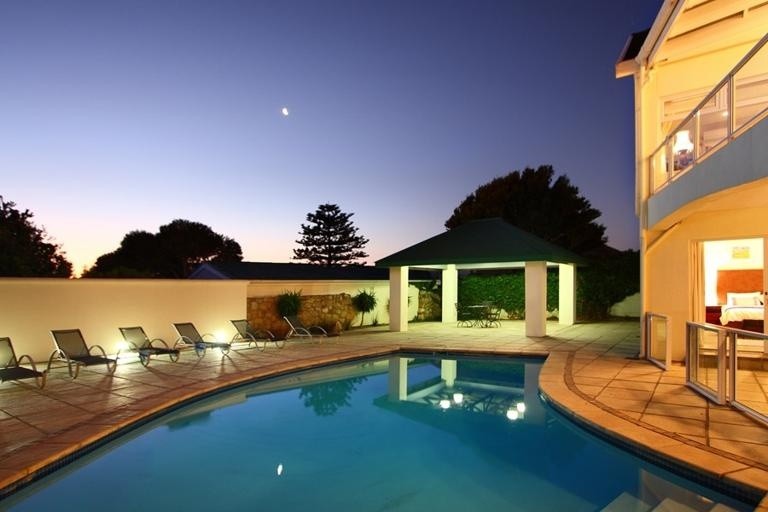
[45,328,117,380]
[283,315,329,348]
[114,325,178,366]
[1,335,46,390]
[229,319,280,352]
[172,321,230,362]
[452,299,503,330]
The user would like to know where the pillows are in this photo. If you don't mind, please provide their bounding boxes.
[727,291,763,307]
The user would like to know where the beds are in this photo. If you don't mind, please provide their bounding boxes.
[717,269,765,339]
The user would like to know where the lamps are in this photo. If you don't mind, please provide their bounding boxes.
[671,130,692,168]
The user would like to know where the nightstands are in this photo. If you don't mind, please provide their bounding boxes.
[705,306,721,325]
